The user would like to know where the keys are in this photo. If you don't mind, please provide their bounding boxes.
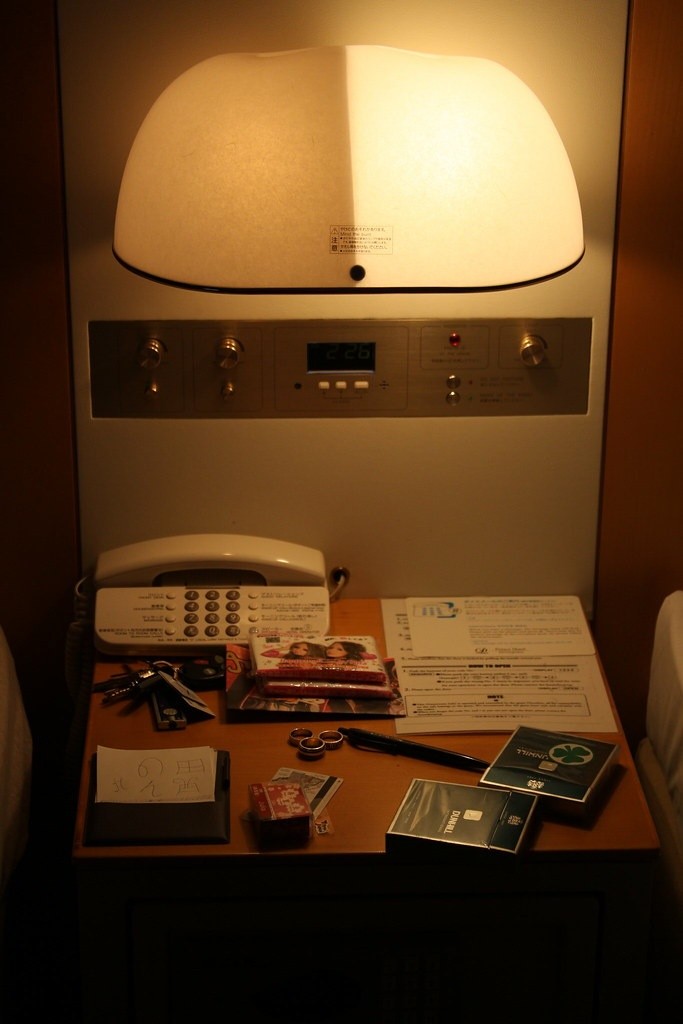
[101,662,174,714]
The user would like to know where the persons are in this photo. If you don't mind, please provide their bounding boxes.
[262,640,376,669]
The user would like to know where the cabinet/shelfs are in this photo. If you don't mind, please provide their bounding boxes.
[72,598,661,1023]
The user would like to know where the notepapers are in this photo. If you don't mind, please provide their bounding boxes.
[94,744,218,805]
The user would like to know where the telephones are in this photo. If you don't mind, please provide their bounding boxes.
[90,532,332,659]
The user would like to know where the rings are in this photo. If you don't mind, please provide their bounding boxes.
[289,728,314,746]
[298,737,326,759]
[318,730,343,749]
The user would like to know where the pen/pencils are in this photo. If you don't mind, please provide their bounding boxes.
[338,727,493,769]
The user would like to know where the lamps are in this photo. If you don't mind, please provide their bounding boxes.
[111,43,586,296]
